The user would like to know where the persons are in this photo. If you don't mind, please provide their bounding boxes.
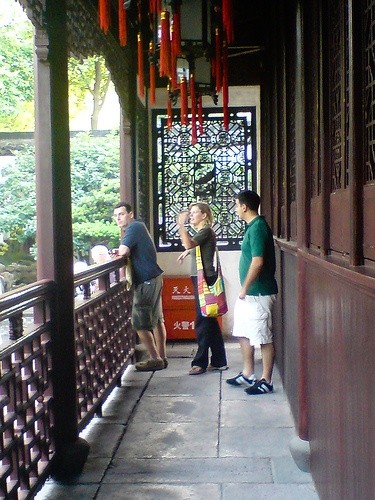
[177,203,229,374]
[226,191,278,394]
[74,245,114,300]
[109,201,168,370]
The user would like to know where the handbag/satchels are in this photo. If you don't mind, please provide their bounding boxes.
[197,263,228,318]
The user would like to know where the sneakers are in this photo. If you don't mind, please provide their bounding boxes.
[226,372,257,387]
[245,378,273,394]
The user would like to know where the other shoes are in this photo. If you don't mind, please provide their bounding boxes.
[189,366,205,374]
[206,363,228,370]
[162,358,169,368]
[136,358,164,370]
